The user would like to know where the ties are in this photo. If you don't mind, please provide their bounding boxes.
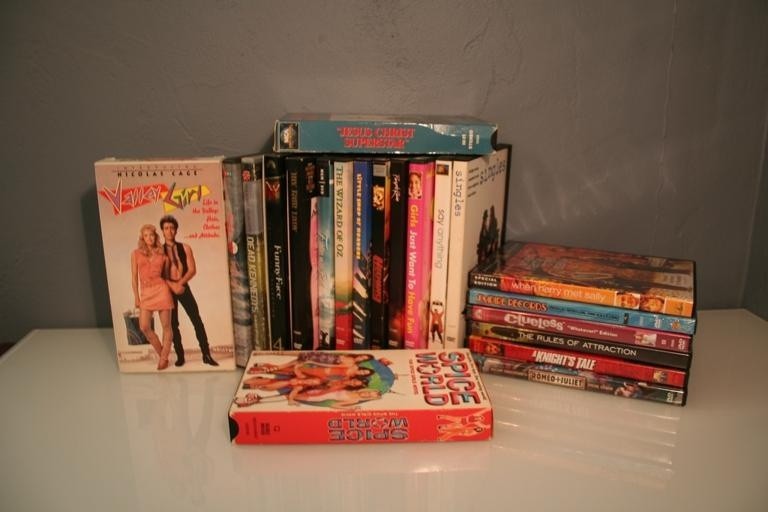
[171,243,178,268]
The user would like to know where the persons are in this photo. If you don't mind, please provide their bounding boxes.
[615,292,640,309]
[131,225,175,370]
[233,352,374,408]
[430,309,442,344]
[640,297,665,312]
[160,216,218,366]
[408,172,421,199]
[477,206,497,263]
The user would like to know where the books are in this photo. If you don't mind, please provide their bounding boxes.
[94,155,236,373]
[464,239,697,407]
[223,111,513,368]
[227,349,494,445]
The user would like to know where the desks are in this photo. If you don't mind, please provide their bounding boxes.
[0,305,767,512]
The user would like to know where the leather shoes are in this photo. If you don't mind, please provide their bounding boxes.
[175,358,184,365]
[204,355,218,365]
[158,359,168,370]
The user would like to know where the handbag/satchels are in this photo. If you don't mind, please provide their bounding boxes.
[123,307,153,344]
